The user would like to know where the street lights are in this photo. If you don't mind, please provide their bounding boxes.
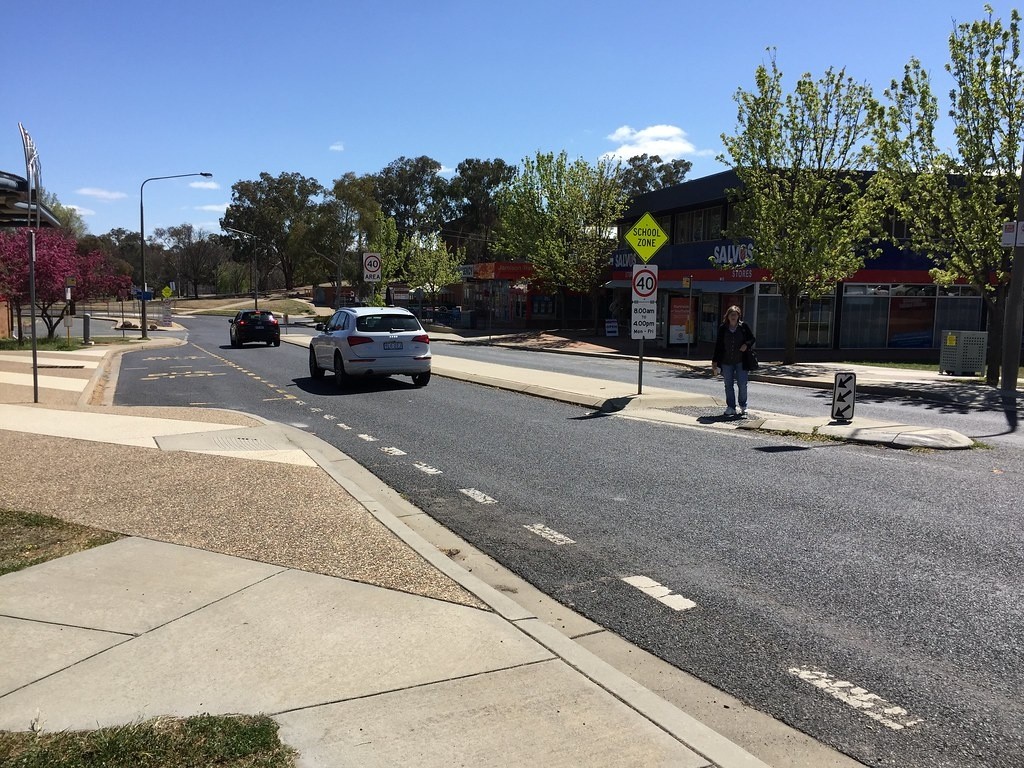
[139,172,213,345]
[224,227,259,310]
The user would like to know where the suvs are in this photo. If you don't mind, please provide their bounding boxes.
[227,309,281,349]
[308,305,432,388]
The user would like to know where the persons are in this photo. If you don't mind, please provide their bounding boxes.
[712,306,756,419]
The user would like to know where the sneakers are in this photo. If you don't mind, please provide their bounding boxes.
[725,407,736,415]
[742,409,749,415]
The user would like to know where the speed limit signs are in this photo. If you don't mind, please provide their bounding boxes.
[363,252,381,282]
[632,263,658,303]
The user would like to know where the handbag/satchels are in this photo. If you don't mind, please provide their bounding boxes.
[742,349,759,371]
